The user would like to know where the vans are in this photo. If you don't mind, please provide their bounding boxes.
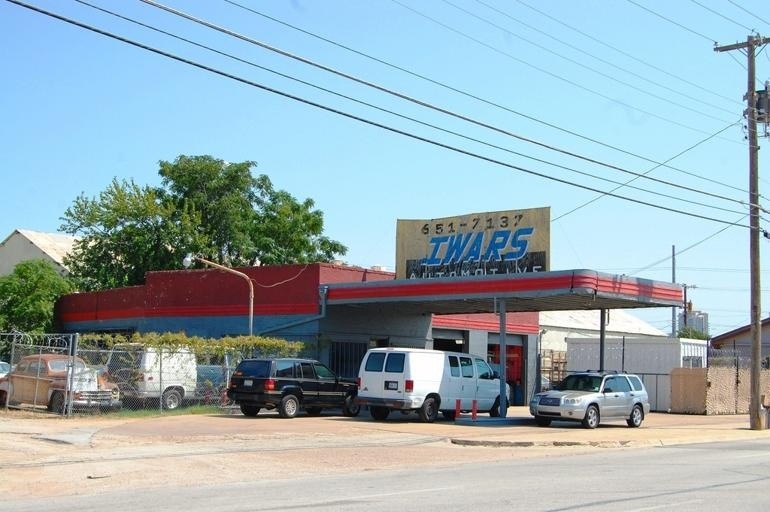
[106,343,245,410]
[357,347,510,422]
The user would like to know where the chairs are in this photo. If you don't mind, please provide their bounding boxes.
[582,378,593,391]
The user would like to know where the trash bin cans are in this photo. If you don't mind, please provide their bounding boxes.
[749,394,770,430]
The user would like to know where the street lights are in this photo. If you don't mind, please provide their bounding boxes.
[182,253,254,336]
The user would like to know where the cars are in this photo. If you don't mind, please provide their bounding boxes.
[529,370,650,429]
[0,354,122,414]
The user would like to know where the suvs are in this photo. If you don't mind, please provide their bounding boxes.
[227,358,361,418]
